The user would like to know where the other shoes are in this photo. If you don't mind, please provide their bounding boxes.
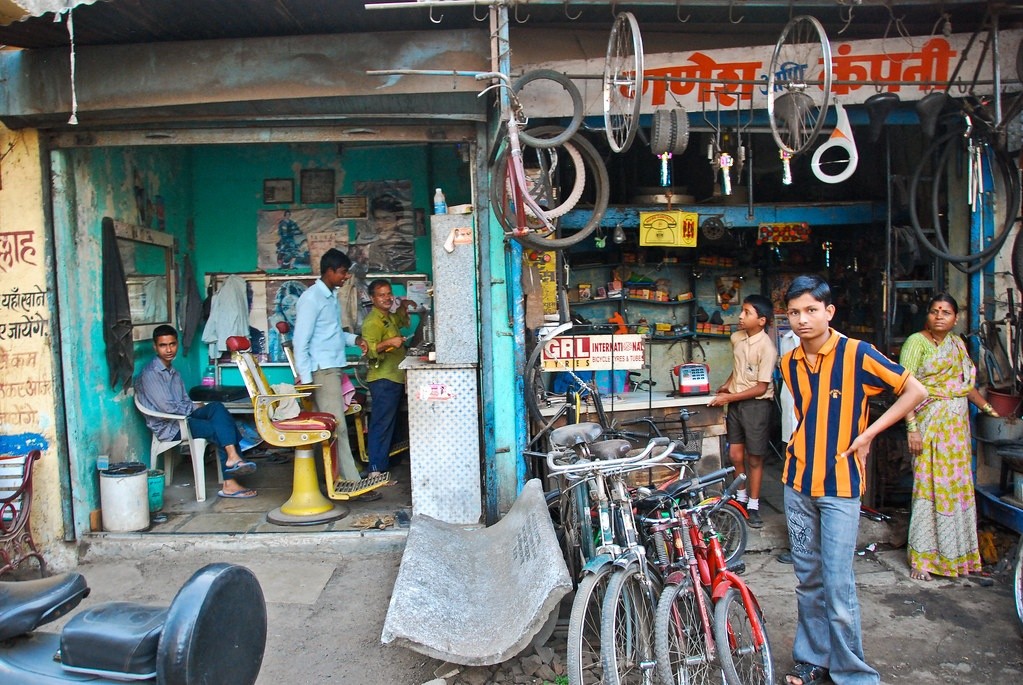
[743,507,764,528]
[350,490,383,502]
[733,498,749,509]
[776,551,796,564]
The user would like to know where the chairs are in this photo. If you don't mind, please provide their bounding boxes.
[227,335,391,525]
[282,340,409,464]
[133,392,225,503]
[0,450,48,579]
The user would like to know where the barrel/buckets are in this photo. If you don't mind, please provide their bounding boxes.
[975,400,1023,441]
[147,468,165,512]
[99,462,150,534]
[984,386,1020,417]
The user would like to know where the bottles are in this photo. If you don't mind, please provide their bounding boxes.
[434,188,446,215]
[202,367,215,385]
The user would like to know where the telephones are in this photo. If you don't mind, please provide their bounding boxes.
[674,363,710,397]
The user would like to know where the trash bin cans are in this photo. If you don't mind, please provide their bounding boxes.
[99,461,150,532]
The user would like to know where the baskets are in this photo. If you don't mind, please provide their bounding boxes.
[667,429,704,456]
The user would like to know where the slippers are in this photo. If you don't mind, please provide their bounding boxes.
[782,661,830,685]
[223,460,258,477]
[217,485,258,498]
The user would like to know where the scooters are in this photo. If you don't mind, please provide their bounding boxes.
[0,562,268,685]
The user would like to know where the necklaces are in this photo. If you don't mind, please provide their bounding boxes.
[931,333,938,346]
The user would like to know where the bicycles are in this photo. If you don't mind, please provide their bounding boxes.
[521,378,774,685]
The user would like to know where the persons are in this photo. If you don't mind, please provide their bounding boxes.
[707,294,778,527]
[899,293,1000,580]
[776,330,799,563]
[135,325,258,497]
[247,283,265,353]
[783,276,929,685]
[291,249,382,501]
[361,280,416,486]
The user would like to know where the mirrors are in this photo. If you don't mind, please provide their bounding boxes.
[105,217,177,342]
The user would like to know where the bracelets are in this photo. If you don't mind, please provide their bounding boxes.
[983,403,994,413]
[906,421,917,431]
[907,417,915,422]
